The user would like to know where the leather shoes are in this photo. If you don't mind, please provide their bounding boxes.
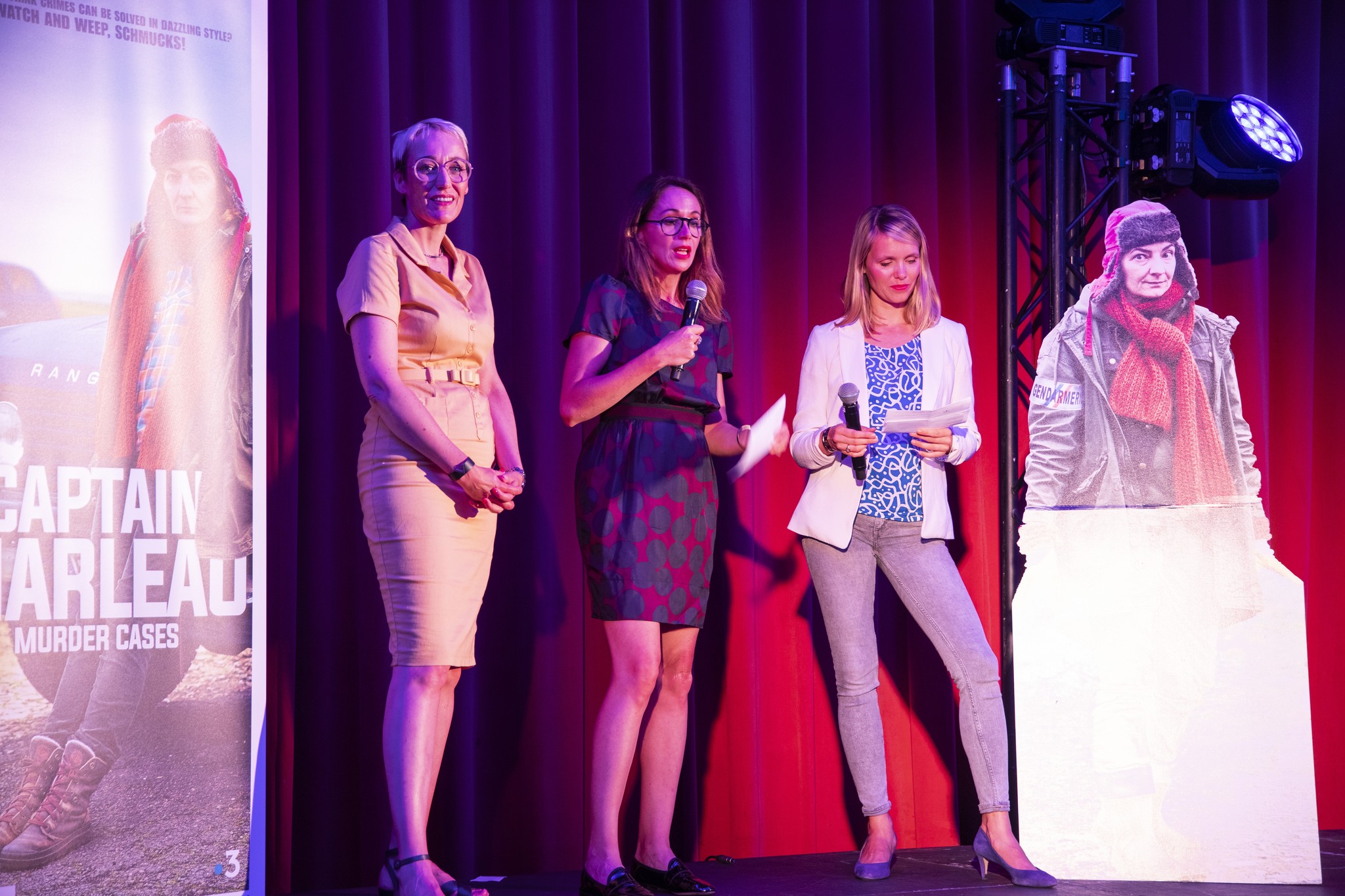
[579,864,655,896]
[630,852,716,895]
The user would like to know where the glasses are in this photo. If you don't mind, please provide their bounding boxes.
[400,158,474,183]
[639,216,711,238]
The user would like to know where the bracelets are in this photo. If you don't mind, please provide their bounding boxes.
[447,456,476,482]
[822,426,837,453]
[736,424,752,451]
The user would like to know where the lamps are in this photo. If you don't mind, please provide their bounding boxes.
[1213,93,1304,174]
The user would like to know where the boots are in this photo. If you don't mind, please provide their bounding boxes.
[0,734,66,853]
[0,739,111,871]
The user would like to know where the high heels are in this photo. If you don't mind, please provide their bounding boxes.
[854,832,898,881]
[973,826,1058,887]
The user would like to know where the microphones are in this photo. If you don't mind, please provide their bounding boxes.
[669,280,707,382]
[837,383,867,480]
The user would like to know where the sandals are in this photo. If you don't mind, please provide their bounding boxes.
[378,847,490,896]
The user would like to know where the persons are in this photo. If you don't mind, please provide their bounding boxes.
[786,202,1059,888]
[0,114,253,874]
[328,117,526,895]
[562,171,791,896]
[1025,198,1264,511]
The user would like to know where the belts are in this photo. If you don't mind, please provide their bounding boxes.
[397,368,481,387]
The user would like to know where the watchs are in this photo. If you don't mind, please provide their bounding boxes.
[505,467,526,489]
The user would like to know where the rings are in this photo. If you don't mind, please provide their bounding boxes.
[846,444,850,453]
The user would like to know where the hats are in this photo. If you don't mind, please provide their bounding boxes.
[1083,199,1200,356]
[142,112,249,242]
